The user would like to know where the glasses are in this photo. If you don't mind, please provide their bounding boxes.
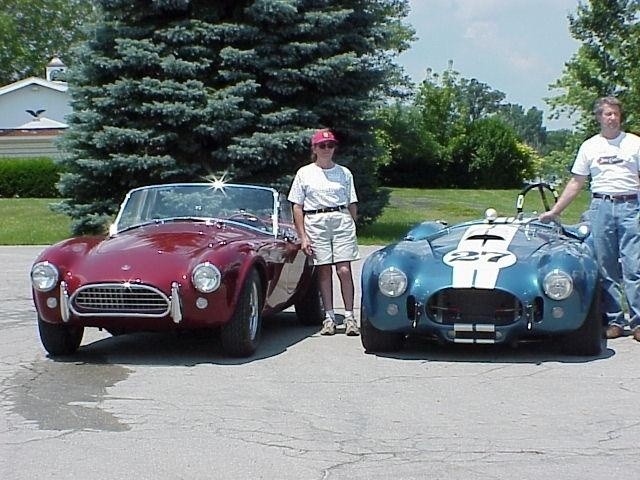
[319,142,334,149]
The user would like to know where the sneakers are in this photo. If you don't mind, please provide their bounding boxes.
[320,317,336,335]
[633,328,640,341]
[343,316,360,336]
[603,325,624,337]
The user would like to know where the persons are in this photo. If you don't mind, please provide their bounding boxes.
[287,131,363,336]
[538,96,640,342]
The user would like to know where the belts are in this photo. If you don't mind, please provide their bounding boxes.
[593,194,637,201]
[307,205,344,214]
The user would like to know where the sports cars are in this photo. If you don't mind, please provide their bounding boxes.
[360,183,608,356]
[31,183,326,355]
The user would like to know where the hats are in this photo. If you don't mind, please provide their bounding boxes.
[312,131,338,145]
